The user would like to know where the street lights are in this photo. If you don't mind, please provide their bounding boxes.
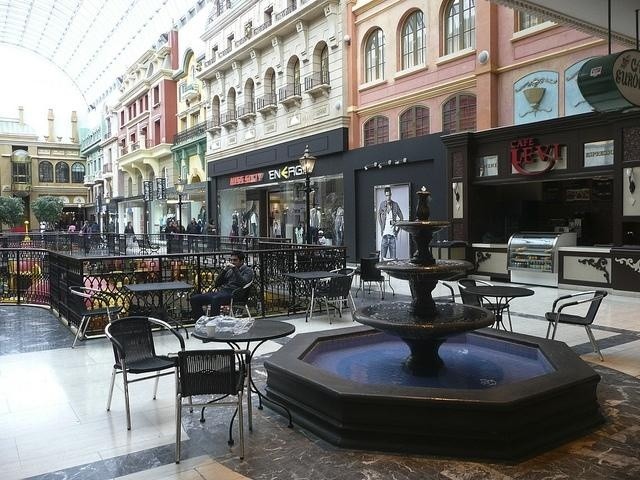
[174,175,186,232]
[105,193,111,231]
[300,145,316,245]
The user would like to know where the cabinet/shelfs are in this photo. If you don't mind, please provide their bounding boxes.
[507,231,578,287]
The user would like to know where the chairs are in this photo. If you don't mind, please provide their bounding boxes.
[545,290,608,362]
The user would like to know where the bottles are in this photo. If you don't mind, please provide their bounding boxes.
[510,259,551,269]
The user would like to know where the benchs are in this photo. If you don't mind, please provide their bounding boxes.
[137,239,160,255]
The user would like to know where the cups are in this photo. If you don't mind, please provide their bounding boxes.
[205,323,216,337]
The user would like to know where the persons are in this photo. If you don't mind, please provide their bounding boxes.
[189,250,254,326]
[164,217,217,253]
[376,186,403,262]
[67,219,137,250]
[229,206,345,253]
